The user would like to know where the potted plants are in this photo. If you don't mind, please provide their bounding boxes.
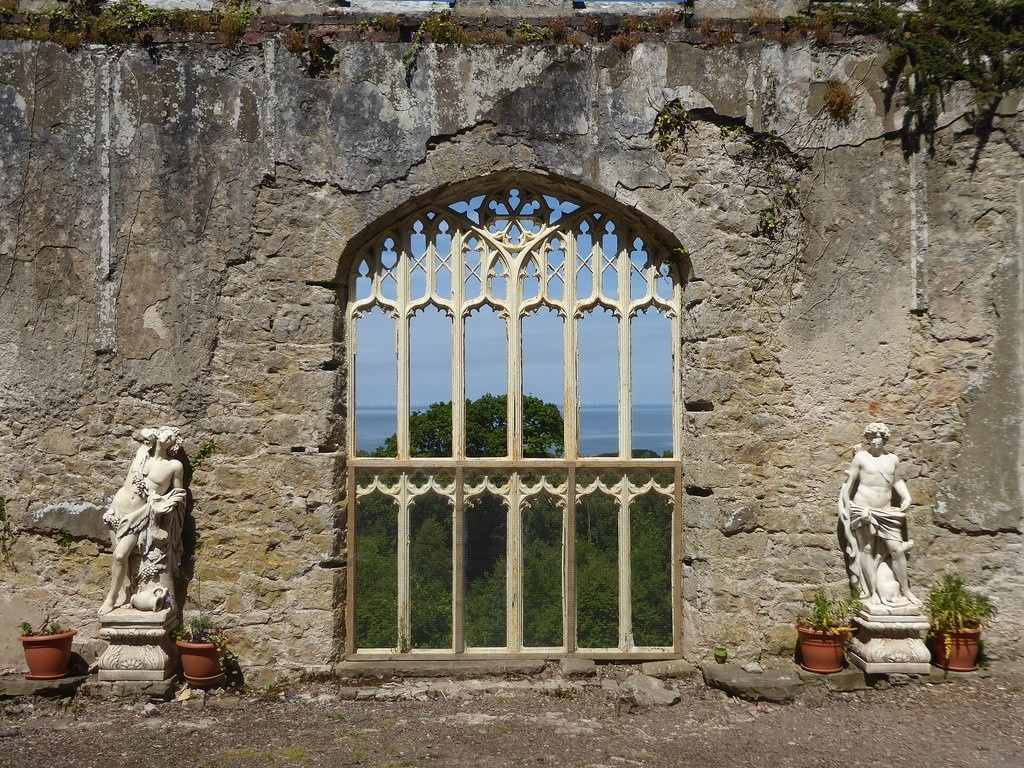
[796,586,861,673]
[171,612,237,687]
[17,618,77,680]
[923,573,999,672]
